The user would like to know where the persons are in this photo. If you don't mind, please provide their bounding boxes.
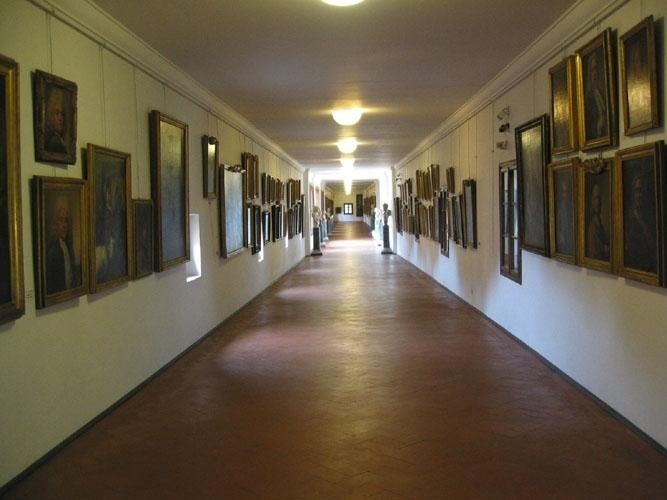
[44,85,66,155]
[45,193,79,294]
[546,23,666,289]
[312,206,333,228]
[370,203,390,225]
